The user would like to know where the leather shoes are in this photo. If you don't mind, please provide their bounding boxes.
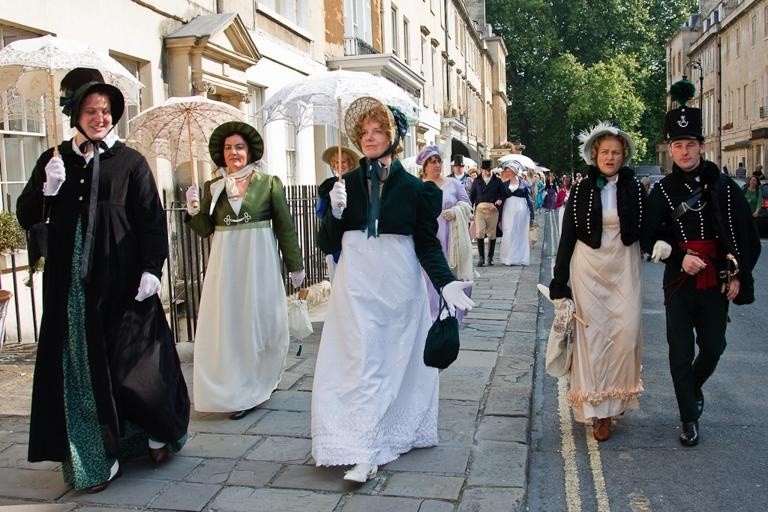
[231,407,254,419]
[343,460,379,482]
[594,418,610,440]
[87,464,122,493]
[681,388,704,446]
[147,444,169,462]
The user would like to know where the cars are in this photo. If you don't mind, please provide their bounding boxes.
[734,178,768,239]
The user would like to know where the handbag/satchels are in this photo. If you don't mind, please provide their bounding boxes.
[546,298,576,377]
[424,294,459,369]
[530,221,539,243]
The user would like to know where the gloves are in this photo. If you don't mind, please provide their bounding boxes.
[43,157,67,196]
[329,179,347,215]
[441,280,476,311]
[134,271,161,302]
[652,241,671,262]
[289,269,306,289]
[185,186,200,217]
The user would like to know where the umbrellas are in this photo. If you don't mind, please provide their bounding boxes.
[123,95,247,208]
[255,65,422,207]
[0,34,145,182]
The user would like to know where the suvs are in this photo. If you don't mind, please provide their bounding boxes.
[632,166,672,193]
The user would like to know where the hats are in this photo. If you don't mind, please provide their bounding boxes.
[345,96,409,161]
[323,145,361,169]
[504,160,520,175]
[468,169,481,176]
[451,156,466,167]
[664,104,702,144]
[481,160,494,169]
[583,121,635,166]
[417,146,440,166]
[208,122,264,166]
[58,67,125,126]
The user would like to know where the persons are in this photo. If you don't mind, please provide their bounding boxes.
[184,131,306,419]
[546,173,584,210]
[416,147,549,324]
[642,178,651,193]
[322,147,359,176]
[549,129,672,440]
[723,162,765,219]
[311,105,476,483]
[16,92,191,494]
[642,140,761,446]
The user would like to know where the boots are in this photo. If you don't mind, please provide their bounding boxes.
[488,239,496,266]
[477,238,485,266]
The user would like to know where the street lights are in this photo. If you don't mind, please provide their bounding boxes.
[672,60,704,105]
